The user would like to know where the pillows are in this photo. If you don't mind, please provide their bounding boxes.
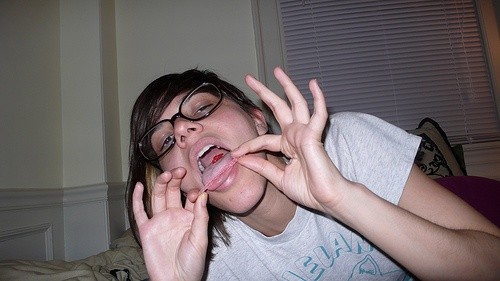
[402,118,463,178]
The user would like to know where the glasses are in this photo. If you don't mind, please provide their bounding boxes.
[136,78,223,165]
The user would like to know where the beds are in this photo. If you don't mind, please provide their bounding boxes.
[23,117,469,281]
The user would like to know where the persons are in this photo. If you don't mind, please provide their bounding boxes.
[123,65,499,281]
